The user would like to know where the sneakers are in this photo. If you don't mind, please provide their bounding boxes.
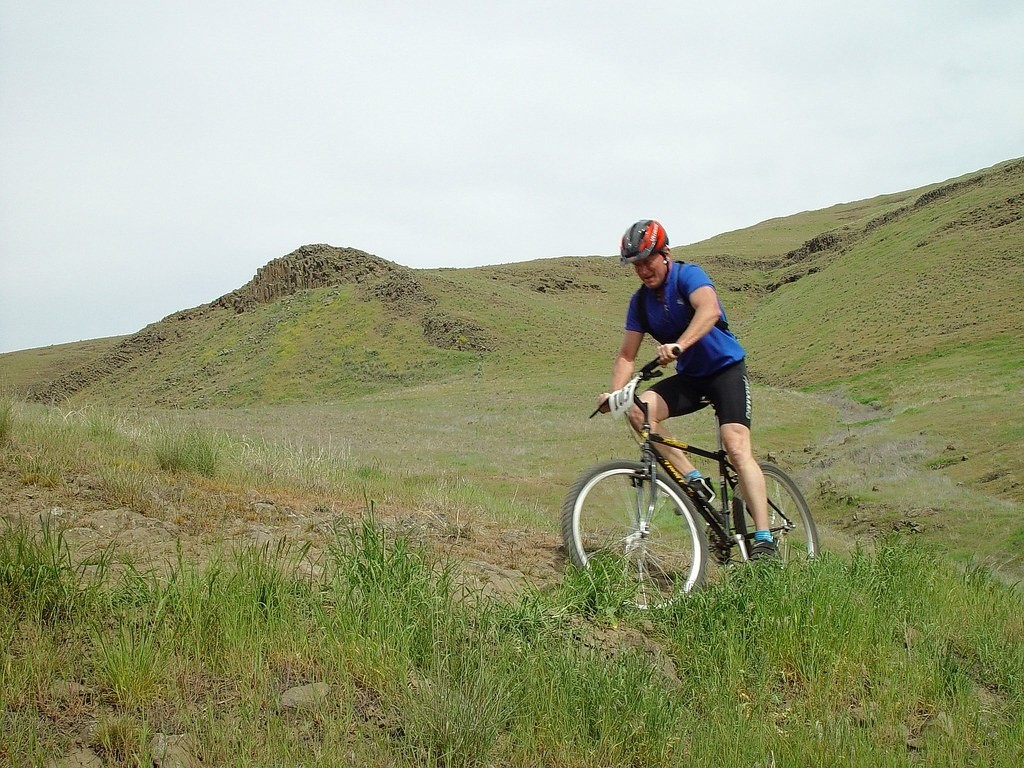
[674,476,715,515]
[751,539,777,563]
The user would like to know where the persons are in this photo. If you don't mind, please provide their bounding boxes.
[596,219,776,562]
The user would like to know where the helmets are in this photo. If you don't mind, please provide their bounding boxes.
[620,220,669,263]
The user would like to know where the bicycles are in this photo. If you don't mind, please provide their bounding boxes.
[562,347,819,611]
